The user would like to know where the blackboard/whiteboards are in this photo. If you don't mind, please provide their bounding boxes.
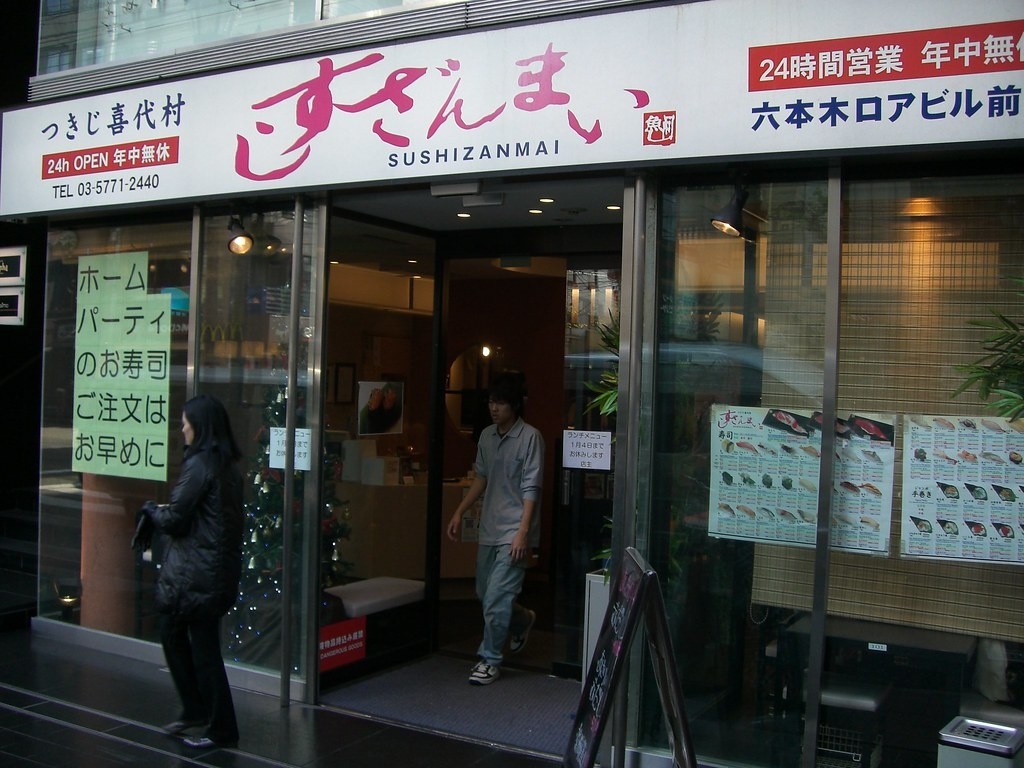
[561,545,654,768]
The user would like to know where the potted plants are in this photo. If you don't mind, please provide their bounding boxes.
[579,291,725,693]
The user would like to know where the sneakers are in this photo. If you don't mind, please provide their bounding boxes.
[510,610,536,653]
[469,661,501,686]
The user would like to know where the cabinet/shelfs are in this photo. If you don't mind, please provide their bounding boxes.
[334,481,480,580]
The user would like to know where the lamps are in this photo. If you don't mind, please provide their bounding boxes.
[710,183,750,237]
[226,216,254,255]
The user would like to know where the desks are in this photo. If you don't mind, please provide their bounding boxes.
[776,613,978,692]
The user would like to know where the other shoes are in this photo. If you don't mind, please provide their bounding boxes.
[183,737,238,749]
[163,719,209,733]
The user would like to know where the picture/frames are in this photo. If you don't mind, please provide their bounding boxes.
[357,381,404,436]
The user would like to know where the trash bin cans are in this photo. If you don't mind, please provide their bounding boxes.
[937,716,1024,768]
[581,566,612,765]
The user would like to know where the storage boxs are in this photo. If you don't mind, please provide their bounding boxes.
[325,574,430,686]
[798,676,890,763]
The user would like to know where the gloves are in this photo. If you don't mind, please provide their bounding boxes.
[131,512,153,553]
[136,500,157,519]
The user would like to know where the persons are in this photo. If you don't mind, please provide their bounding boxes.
[671,503,754,655]
[139,395,245,748]
[442,388,545,686]
